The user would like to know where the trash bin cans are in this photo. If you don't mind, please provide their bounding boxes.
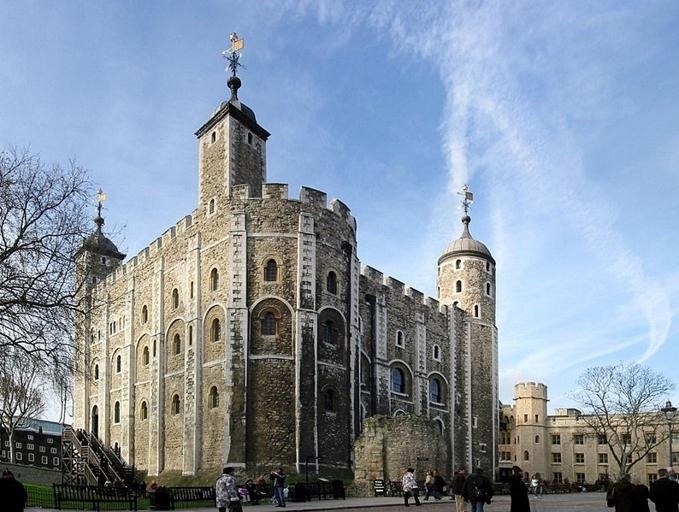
[149,488,170,511]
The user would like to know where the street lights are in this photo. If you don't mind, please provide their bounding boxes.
[661,401,677,467]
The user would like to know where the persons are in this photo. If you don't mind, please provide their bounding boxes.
[508,465,531,512]
[245,478,262,505]
[215,467,242,511]
[424,467,494,511]
[238,484,251,504]
[295,478,312,502]
[520,475,586,494]
[76,427,87,446]
[529,474,539,498]
[269,468,287,507]
[402,468,422,506]
[100,459,108,474]
[605,462,679,511]
[0,470,28,511]
[100,455,108,465]
[256,476,267,498]
[145,478,158,493]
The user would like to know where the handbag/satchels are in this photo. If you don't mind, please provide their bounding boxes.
[411,487,419,497]
[607,496,614,507]
[230,501,242,512]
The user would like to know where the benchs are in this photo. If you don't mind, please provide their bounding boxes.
[387,479,427,498]
[51,482,139,512]
[167,479,349,511]
